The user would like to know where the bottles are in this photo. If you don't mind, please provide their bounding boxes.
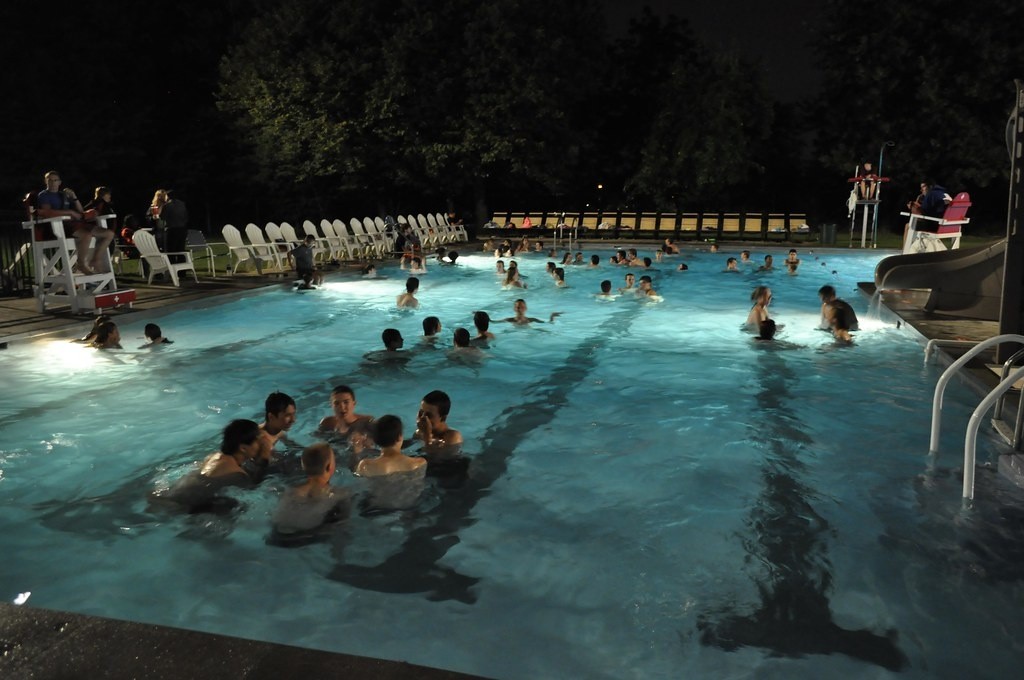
[151,204,159,219]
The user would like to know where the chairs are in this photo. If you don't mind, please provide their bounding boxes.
[858,167,878,187]
[132,230,200,287]
[186,231,233,277]
[265,222,305,271]
[765,214,788,242]
[397,214,467,247]
[374,216,396,253]
[244,223,294,274]
[222,224,275,275]
[476,212,763,243]
[899,192,972,255]
[332,219,364,259]
[22,190,116,313]
[788,214,810,242]
[350,218,380,259]
[279,222,319,271]
[302,219,334,262]
[113,239,141,276]
[320,220,361,261]
[363,217,389,257]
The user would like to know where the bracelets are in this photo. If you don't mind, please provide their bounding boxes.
[72,198,78,201]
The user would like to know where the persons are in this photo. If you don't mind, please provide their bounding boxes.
[287,234,325,290]
[199,418,273,490]
[354,415,427,476]
[34,170,115,275]
[319,385,373,434]
[92,321,123,349]
[83,186,117,256]
[358,161,952,363]
[277,442,337,499]
[253,392,306,457]
[119,189,188,279]
[79,315,111,341]
[410,389,463,445]
[141,323,174,345]
